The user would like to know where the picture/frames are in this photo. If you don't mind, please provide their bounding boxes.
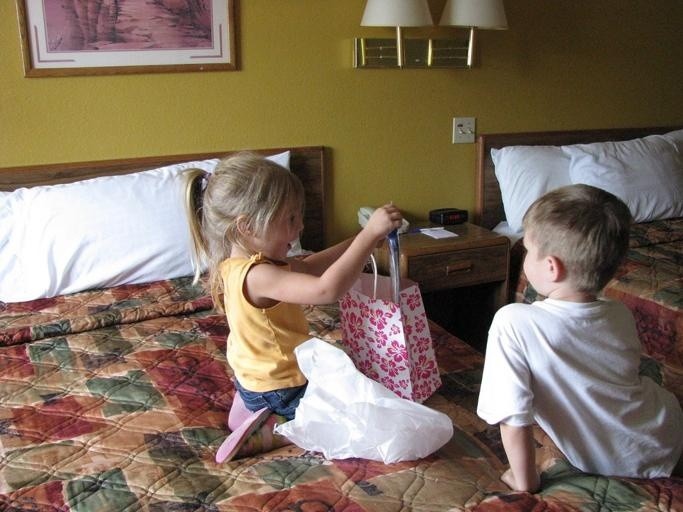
[12,1,239,79]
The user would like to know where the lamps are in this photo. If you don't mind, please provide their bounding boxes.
[353,1,509,71]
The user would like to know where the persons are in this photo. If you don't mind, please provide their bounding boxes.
[476,184,682,493]
[174,151,402,465]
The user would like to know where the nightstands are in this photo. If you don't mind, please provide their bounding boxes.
[373,220,511,306]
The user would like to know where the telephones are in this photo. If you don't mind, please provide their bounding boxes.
[358,206,410,238]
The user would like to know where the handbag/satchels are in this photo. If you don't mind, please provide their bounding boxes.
[338,272,443,404]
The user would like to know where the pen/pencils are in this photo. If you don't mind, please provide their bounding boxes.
[415,227,445,232]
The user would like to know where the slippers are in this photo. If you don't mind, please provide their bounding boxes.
[215,389,272,464]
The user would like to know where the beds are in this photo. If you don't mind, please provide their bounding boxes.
[0,127,682,511]
